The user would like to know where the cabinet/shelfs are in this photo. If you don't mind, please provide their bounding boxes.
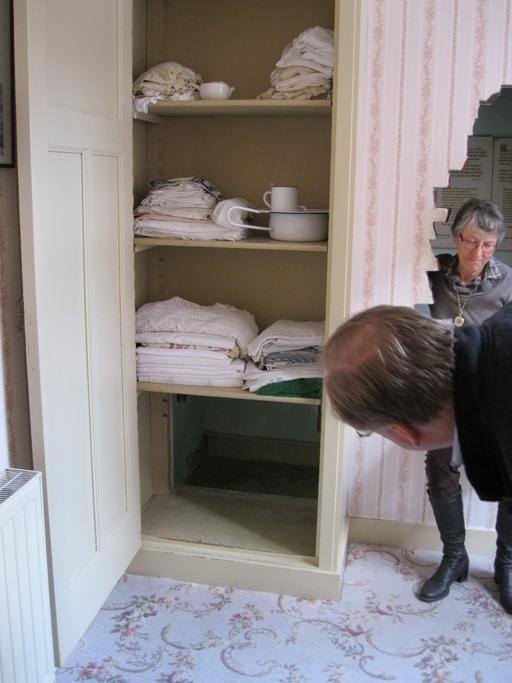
[13,0,351,671]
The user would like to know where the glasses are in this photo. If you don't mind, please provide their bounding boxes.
[458,231,499,254]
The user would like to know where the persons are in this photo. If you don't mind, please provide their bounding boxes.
[419,199,512,615]
[324,305,512,501]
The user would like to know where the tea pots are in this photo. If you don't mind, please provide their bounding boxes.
[191,82,236,100]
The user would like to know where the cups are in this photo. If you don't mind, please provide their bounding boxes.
[264,186,300,212]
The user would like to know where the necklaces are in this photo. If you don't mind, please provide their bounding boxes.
[449,281,479,326]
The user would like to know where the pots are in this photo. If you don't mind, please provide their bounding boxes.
[227,206,328,240]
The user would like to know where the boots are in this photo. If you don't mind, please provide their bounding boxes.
[492,496,512,618]
[418,483,470,603]
